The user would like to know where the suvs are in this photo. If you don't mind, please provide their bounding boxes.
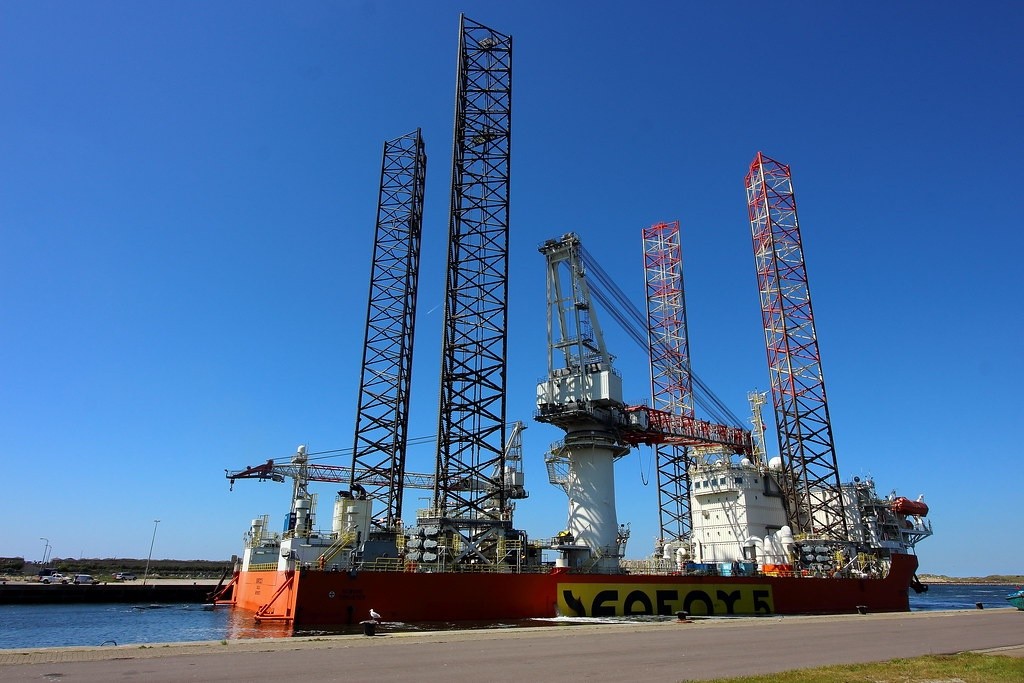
[73,574,100,584]
[116,572,138,581]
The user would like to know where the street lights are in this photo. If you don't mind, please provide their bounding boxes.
[40,537,49,571]
[143,520,161,585]
[45,545,52,565]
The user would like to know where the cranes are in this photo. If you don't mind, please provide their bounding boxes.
[224,8,933,581]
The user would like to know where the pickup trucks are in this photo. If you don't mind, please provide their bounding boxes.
[39,573,72,585]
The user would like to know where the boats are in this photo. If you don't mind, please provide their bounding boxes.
[1005,590,1024,610]
[205,387,936,636]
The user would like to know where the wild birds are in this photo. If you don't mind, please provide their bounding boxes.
[369,609,382,620]
